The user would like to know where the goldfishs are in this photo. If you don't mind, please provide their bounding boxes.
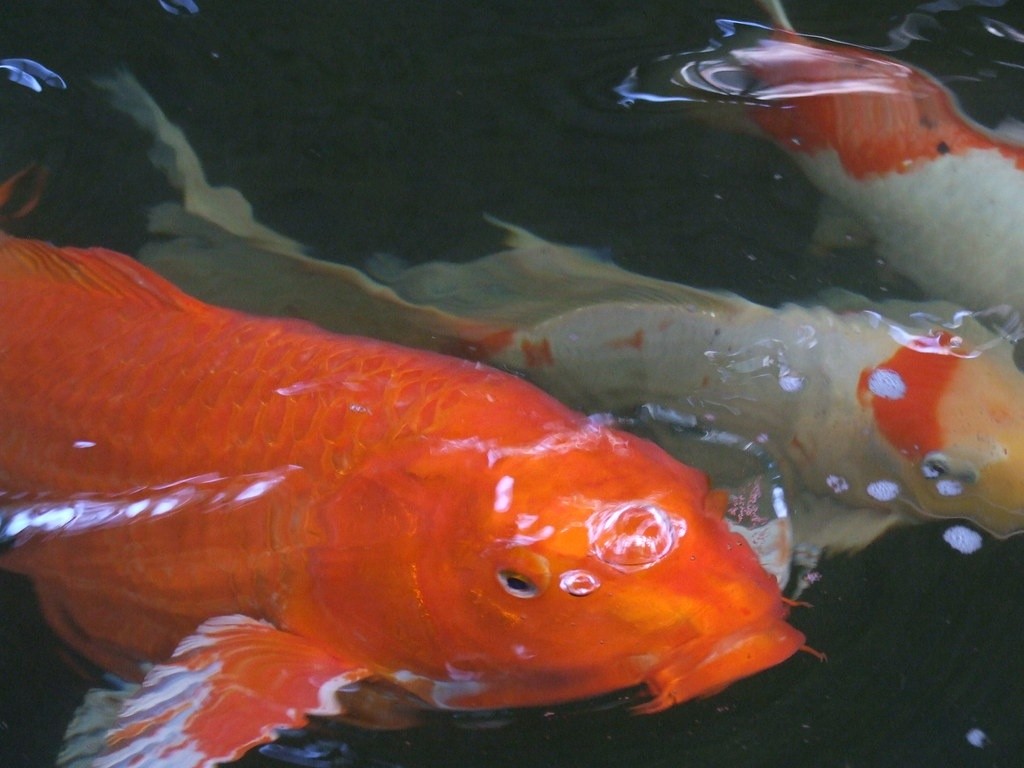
[1,175,824,768]
[109,66,1024,601]
[617,0,1024,345]
